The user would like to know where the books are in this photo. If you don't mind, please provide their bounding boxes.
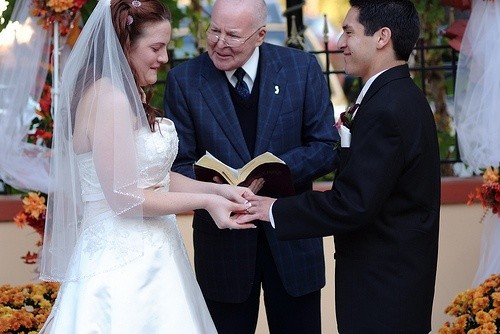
[193,149,289,196]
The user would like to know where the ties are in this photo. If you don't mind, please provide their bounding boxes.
[233,66,251,96]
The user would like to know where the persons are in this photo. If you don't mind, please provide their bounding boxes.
[234,1,442,334]
[64,0,259,334]
[163,1,341,334]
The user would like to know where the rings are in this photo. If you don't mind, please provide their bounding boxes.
[246,208,251,214]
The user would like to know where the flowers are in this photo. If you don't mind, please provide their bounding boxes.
[438,273,500,334]
[0,0,86,334]
[466,161,500,225]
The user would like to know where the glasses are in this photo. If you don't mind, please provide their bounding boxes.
[205,24,265,48]
